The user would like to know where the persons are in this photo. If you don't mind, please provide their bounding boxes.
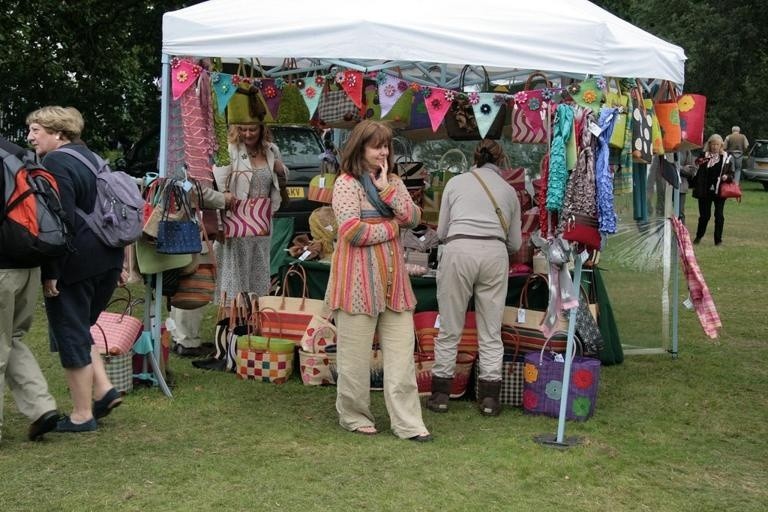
[426,139,523,416]
[687,134,735,245]
[171,183,238,356]
[0,138,59,440]
[212,122,290,319]
[722,126,749,186]
[324,120,433,442]
[26,106,147,432]
[647,149,695,247]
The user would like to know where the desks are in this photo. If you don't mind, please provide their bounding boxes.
[278,255,596,322]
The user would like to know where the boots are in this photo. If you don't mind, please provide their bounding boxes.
[479,378,501,416]
[427,377,453,413]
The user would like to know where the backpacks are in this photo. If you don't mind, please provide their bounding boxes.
[43,148,143,248]
[0,148,79,255]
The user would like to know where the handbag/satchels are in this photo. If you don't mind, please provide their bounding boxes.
[306,157,337,204]
[415,272,601,423]
[389,136,468,228]
[220,169,272,237]
[563,212,602,249]
[720,181,743,198]
[606,78,707,166]
[225,57,508,141]
[658,159,679,188]
[121,177,217,310]
[511,72,558,144]
[215,263,384,391]
[90,287,142,356]
[90,323,133,394]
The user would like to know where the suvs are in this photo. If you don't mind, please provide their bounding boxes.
[112,123,333,237]
[742,139,767,190]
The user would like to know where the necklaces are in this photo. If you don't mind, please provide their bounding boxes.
[247,145,259,157]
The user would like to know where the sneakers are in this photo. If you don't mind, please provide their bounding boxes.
[173,342,216,356]
[29,387,121,441]
